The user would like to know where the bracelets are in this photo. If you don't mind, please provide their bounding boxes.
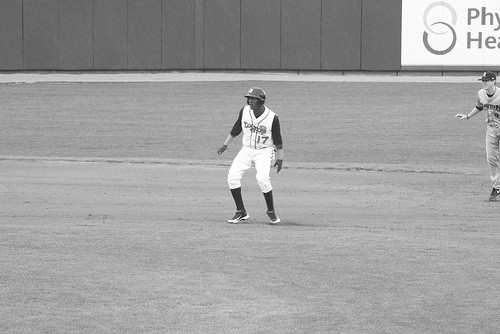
[467,114,469,119]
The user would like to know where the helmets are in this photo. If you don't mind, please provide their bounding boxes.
[245,87,265,105]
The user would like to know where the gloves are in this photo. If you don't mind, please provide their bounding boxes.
[274,159,282,173]
[217,146,226,154]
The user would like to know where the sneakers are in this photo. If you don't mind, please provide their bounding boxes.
[228,210,249,223]
[267,211,280,223]
[489,188,500,200]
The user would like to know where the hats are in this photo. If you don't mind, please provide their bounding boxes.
[478,72,496,80]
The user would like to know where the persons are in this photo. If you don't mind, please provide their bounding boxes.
[454,71,500,202]
[217,87,285,225]
